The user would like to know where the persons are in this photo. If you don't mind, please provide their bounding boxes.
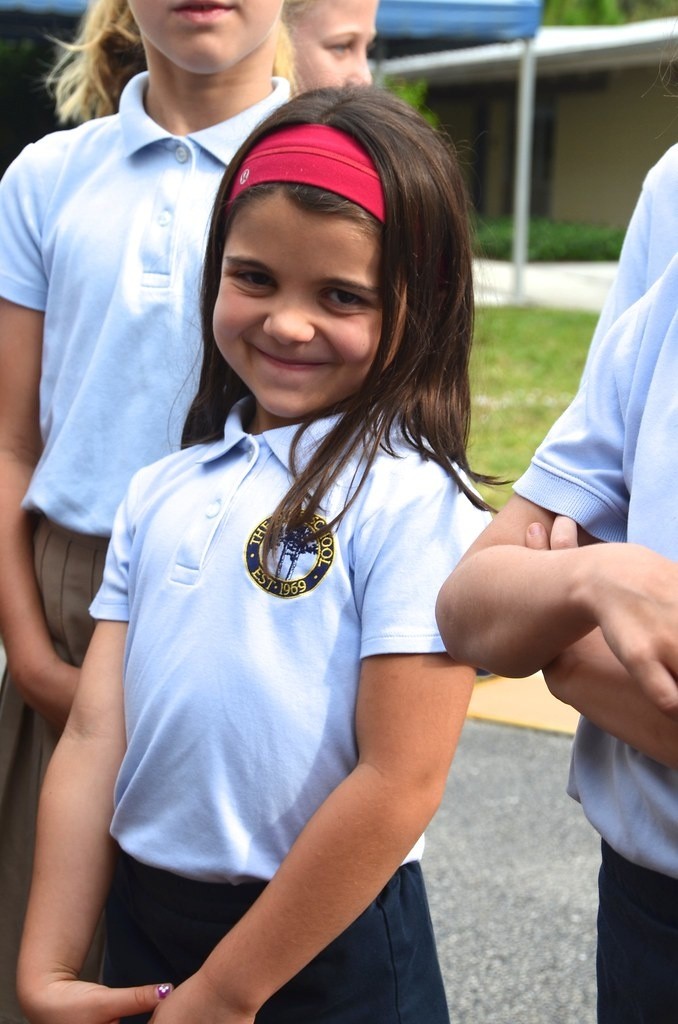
[434,141,678,1024]
[282,0,379,92]
[0,0,302,1024]
[14,85,512,1024]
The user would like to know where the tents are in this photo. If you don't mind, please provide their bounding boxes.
[0,0,541,294]
